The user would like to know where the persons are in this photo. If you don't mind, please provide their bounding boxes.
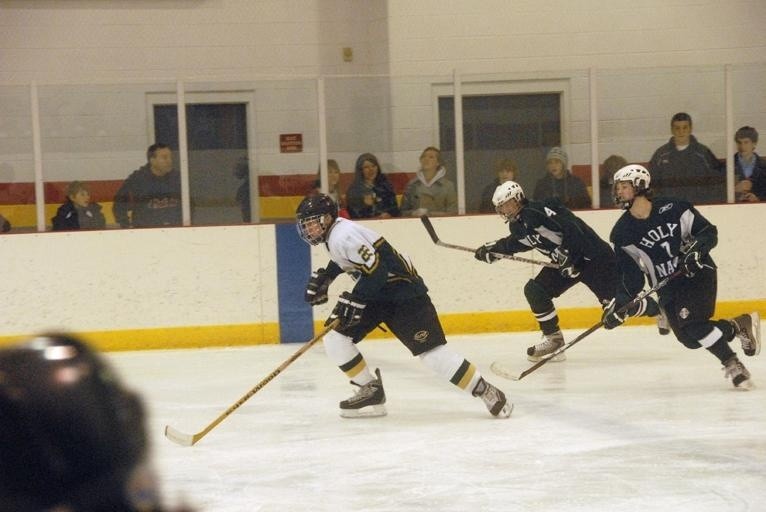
[720,125,766,204]
[398,147,456,215]
[601,163,763,397]
[532,146,593,209]
[316,158,348,219]
[231,155,249,224]
[599,154,631,209]
[294,190,516,423]
[49,181,107,230]
[473,180,673,366]
[648,112,719,203]
[111,141,196,228]
[344,152,400,218]
[0,327,203,512]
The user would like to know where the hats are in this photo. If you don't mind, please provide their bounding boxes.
[544,146,569,170]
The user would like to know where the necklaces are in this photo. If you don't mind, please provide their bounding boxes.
[479,157,521,213]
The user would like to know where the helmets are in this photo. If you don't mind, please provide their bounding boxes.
[491,180,527,207]
[611,165,656,192]
[294,193,338,247]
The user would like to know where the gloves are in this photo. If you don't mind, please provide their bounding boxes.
[473,239,501,265]
[303,267,330,307]
[598,296,626,331]
[323,290,370,332]
[676,239,719,284]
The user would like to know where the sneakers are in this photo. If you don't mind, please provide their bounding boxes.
[469,375,509,420]
[727,310,757,358]
[526,335,566,358]
[338,367,388,411]
[720,352,753,389]
[652,305,673,338]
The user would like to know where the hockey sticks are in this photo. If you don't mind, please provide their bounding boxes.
[165,318,340,446]
[420,214,560,270]
[490,267,681,380]
